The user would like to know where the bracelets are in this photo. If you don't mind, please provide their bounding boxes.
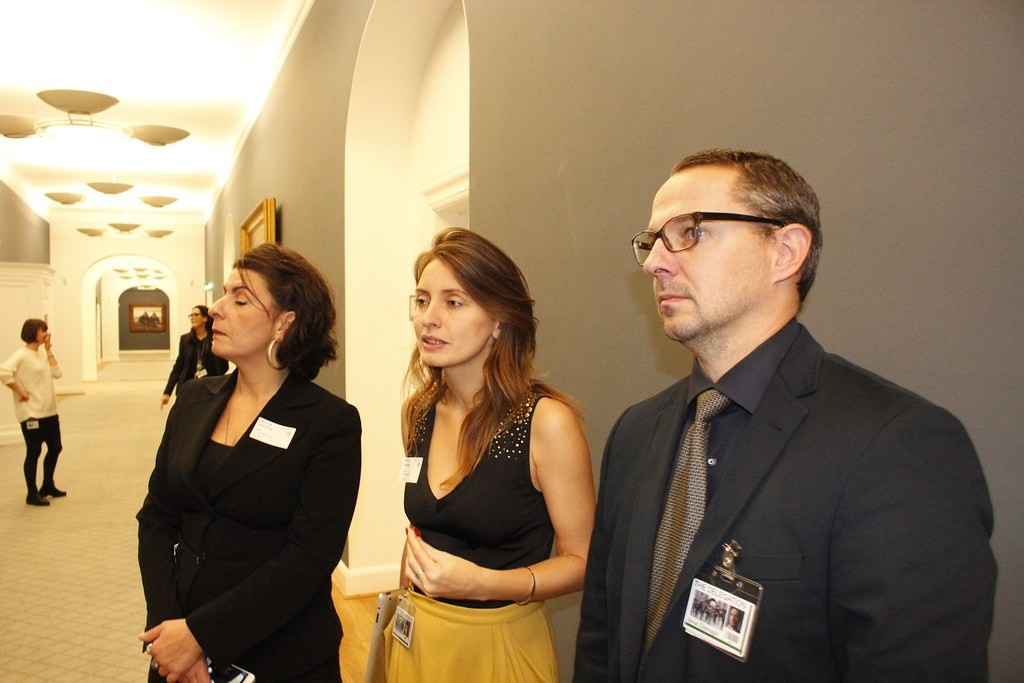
[48,355,54,358]
[514,566,536,606]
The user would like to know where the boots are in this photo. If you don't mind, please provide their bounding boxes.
[40,480,67,497]
[26,487,50,506]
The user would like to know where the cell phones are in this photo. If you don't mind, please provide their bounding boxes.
[206,656,255,683]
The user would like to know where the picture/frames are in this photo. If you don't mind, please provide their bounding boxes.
[129,303,165,332]
[241,198,277,257]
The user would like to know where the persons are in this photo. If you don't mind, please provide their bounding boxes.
[726,607,740,633]
[404,624,408,637]
[0,319,67,507]
[571,148,998,683]
[160,305,229,411]
[134,242,362,683]
[382,227,597,683]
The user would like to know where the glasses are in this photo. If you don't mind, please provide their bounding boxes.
[188,313,203,318]
[630,210,786,267]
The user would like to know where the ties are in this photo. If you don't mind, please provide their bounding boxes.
[641,389,734,682]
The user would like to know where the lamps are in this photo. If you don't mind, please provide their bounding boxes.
[0,89,189,280]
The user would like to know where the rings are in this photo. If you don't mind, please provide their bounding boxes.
[415,568,423,579]
[151,660,158,669]
[146,643,152,655]
